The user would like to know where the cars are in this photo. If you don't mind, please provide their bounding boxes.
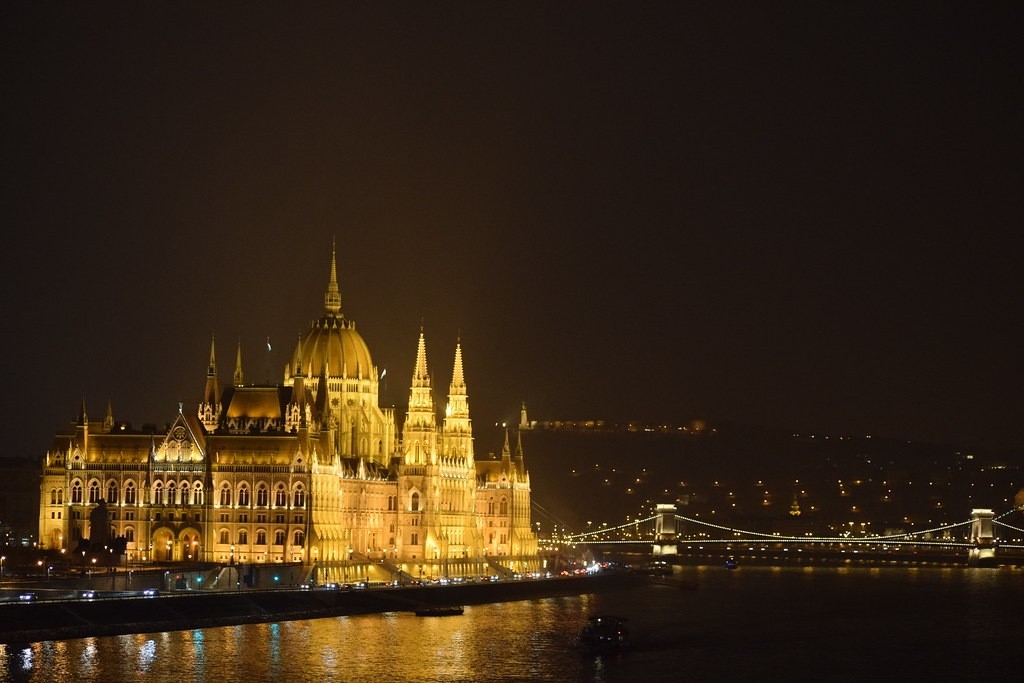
[356,582,368,587]
[386,562,615,588]
[82,591,100,600]
[327,583,339,588]
[143,589,159,596]
[301,584,312,590]
[19,593,37,601]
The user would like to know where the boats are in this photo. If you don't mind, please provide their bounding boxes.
[580,615,630,650]
[412,607,464,617]
